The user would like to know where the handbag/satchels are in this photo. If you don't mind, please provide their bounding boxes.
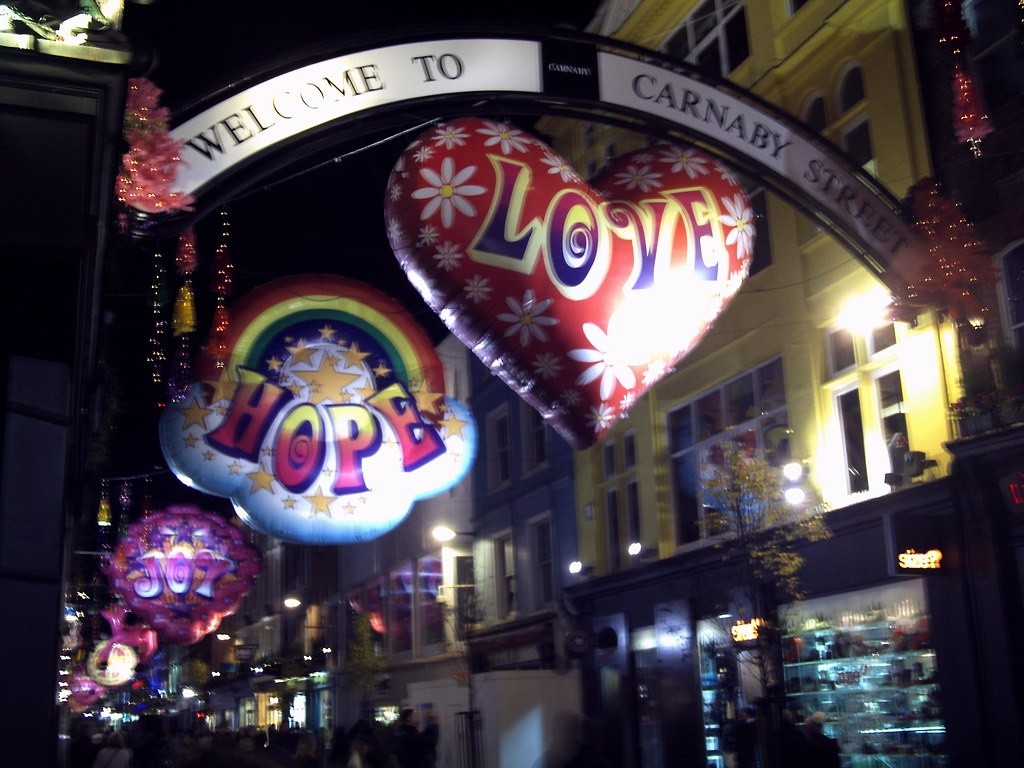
[347,750,362,768]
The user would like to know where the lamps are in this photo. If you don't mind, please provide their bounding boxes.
[629,542,658,561]
[432,527,475,542]
[284,587,308,607]
[568,560,595,576]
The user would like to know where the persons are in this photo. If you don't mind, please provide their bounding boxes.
[65,698,441,768]
[730,705,841,768]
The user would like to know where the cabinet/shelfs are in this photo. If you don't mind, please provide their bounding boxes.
[782,621,947,768]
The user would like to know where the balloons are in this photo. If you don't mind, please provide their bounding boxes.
[108,504,263,647]
[350,556,441,634]
[158,273,476,545]
[68,604,157,712]
[386,117,757,450]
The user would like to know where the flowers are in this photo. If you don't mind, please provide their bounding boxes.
[118,80,197,224]
[888,184,994,331]
